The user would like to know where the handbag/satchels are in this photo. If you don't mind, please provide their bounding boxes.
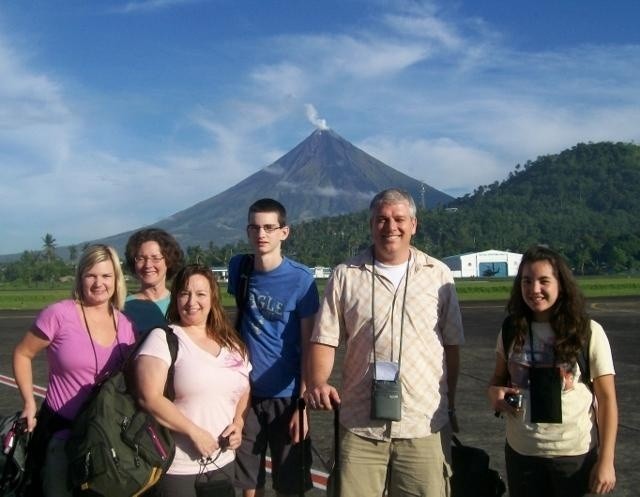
[0,411,57,497]
[446,432,506,497]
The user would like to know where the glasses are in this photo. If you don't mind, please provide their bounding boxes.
[246,223,282,233]
[134,254,166,263]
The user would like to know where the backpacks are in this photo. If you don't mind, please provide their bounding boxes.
[65,325,180,496]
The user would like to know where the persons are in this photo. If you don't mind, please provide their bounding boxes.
[486,245,619,496]
[12,243,140,497]
[121,229,184,336]
[307,187,465,497]
[133,263,253,497]
[227,198,314,497]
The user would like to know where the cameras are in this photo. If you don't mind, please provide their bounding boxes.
[505,393,522,407]
